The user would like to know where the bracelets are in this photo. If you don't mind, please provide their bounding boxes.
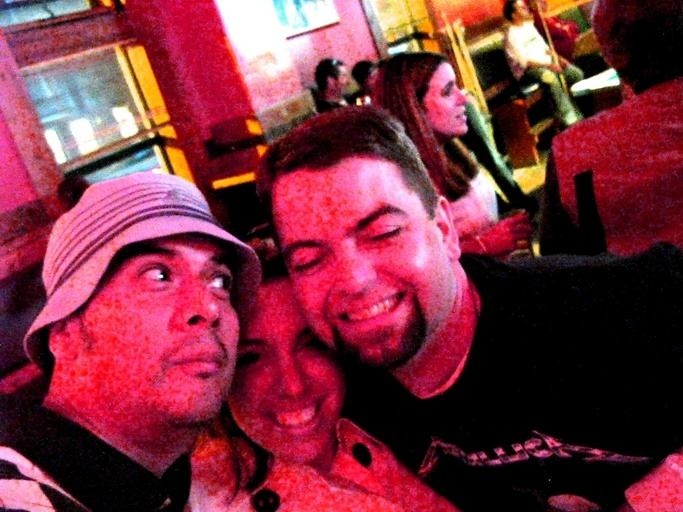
[474,235,489,256]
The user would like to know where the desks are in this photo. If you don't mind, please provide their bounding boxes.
[569,66,622,115]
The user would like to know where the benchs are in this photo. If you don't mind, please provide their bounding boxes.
[492,85,561,170]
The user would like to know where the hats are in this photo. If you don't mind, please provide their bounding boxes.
[22,171,263,375]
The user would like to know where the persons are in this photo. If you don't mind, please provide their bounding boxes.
[374,52,534,261]
[541,0,681,250]
[183,241,460,510]
[259,104,683,512]
[345,61,377,103]
[313,58,350,112]
[503,0,587,130]
[0,172,264,512]
